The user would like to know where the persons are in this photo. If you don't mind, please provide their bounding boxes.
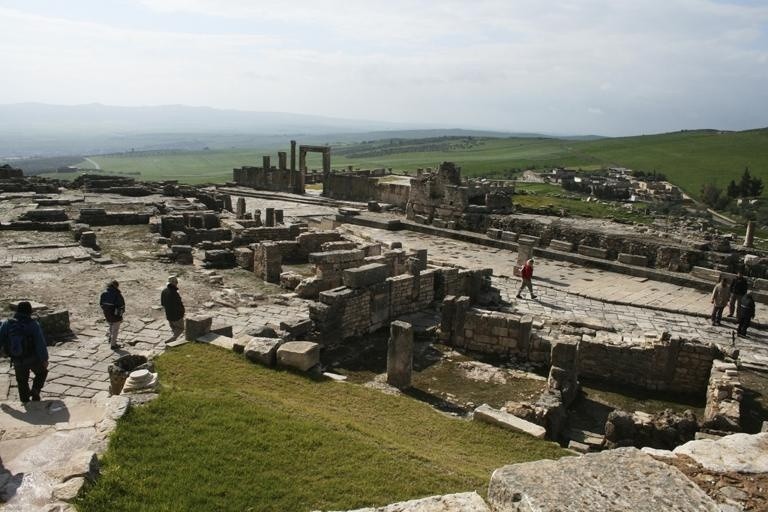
[709,278,731,326]
[0,302,48,402]
[737,290,755,338]
[516,258,537,299]
[99,280,125,350]
[726,270,748,317]
[160,277,185,344]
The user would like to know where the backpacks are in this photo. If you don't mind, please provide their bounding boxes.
[6,319,31,360]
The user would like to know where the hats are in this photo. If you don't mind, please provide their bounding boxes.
[17,302,33,314]
[168,276,177,283]
